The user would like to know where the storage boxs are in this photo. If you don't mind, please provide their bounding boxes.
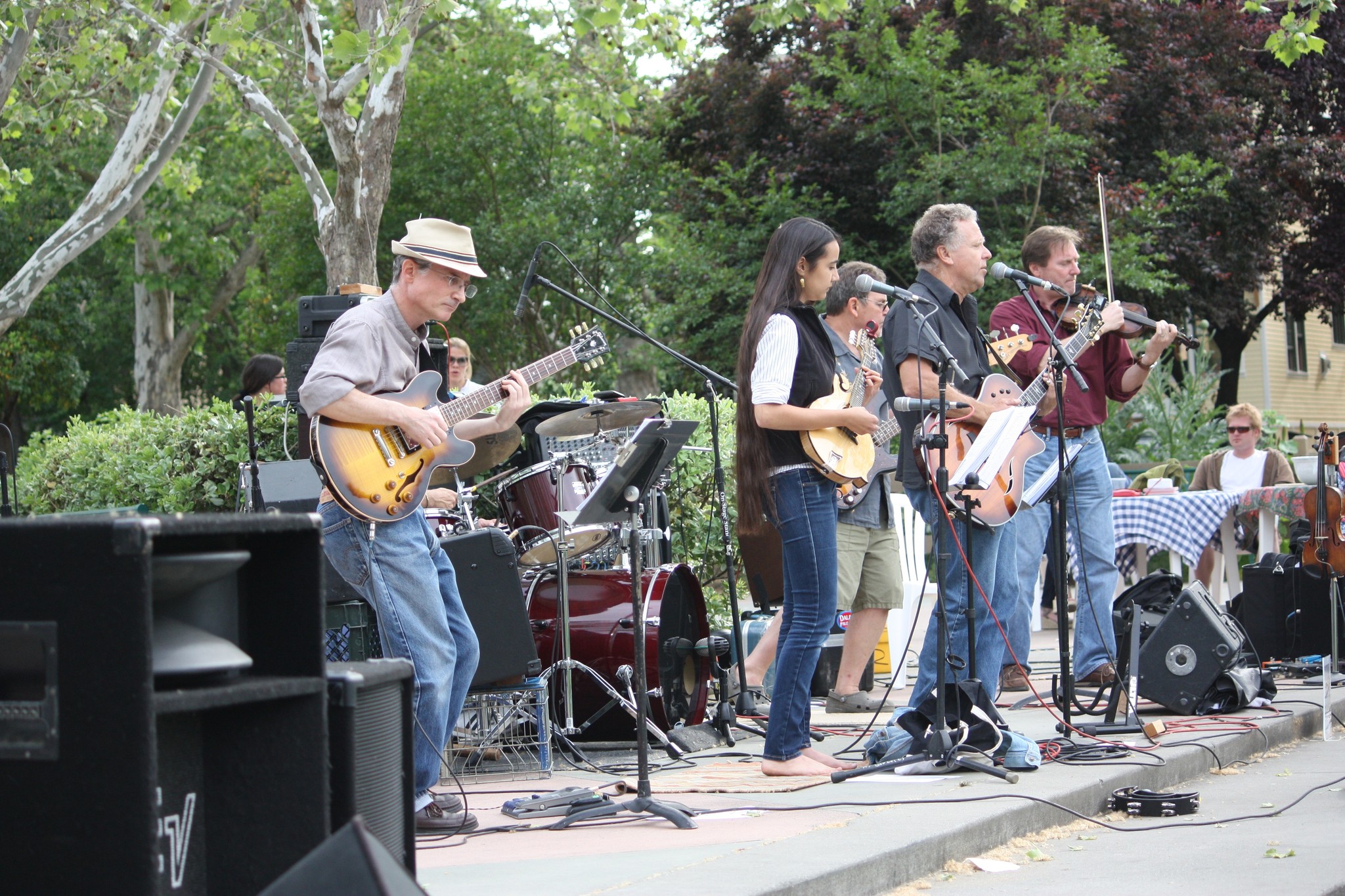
[325,600,384,663]
[810,634,874,696]
[873,629,891,673]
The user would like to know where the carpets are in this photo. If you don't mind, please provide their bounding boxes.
[614,757,870,795]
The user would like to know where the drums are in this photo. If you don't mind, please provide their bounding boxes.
[492,457,614,568]
[522,563,716,740]
[420,507,469,539]
[594,465,643,530]
[497,525,515,548]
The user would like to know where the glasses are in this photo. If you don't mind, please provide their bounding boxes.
[862,296,888,311]
[448,356,469,366]
[1227,426,1250,433]
[273,374,286,380]
[415,262,478,298]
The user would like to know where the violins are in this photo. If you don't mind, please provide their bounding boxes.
[1303,422,1345,579]
[1056,282,1200,351]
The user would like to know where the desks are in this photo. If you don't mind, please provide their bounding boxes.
[1236,485,1345,562]
[1065,489,1275,607]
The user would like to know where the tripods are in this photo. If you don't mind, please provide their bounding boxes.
[547,421,694,834]
[534,272,824,741]
[833,301,1015,782]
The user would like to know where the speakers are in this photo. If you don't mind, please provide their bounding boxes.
[434,527,559,688]
[325,656,419,881]
[1119,577,1245,714]
[0,508,339,896]
[254,815,429,896]
[283,344,448,455]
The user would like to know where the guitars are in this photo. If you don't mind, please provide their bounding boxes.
[800,319,879,489]
[834,389,901,513]
[309,321,611,521]
[912,299,1105,530]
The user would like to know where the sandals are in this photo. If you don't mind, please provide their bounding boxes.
[726,661,772,716]
[825,686,895,714]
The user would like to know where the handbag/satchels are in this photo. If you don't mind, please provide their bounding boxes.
[895,682,1003,776]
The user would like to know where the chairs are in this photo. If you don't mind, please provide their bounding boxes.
[823,492,937,690]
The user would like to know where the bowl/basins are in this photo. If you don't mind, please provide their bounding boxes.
[1290,455,1331,485]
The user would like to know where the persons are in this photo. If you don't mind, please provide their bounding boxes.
[1187,402,1295,590]
[420,487,506,529]
[444,338,487,397]
[735,202,1178,777]
[229,354,287,413]
[297,217,531,835]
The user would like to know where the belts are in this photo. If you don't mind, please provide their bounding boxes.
[1030,421,1095,438]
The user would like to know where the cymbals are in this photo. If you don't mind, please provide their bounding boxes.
[534,401,662,436]
[429,412,522,486]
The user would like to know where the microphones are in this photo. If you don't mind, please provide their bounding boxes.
[514,244,542,317]
[856,274,931,304]
[893,397,967,411]
[991,261,1070,295]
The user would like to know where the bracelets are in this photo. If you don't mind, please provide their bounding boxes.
[425,493,429,508]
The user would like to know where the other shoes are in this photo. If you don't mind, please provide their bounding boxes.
[997,665,1032,690]
[415,801,479,833]
[425,788,463,813]
[1081,664,1120,684]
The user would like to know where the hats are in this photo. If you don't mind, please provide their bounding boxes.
[392,218,487,277]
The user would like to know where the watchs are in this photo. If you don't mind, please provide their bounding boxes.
[1137,354,1157,371]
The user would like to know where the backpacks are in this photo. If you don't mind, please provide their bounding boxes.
[1127,458,1188,493]
[1113,569,1183,606]
